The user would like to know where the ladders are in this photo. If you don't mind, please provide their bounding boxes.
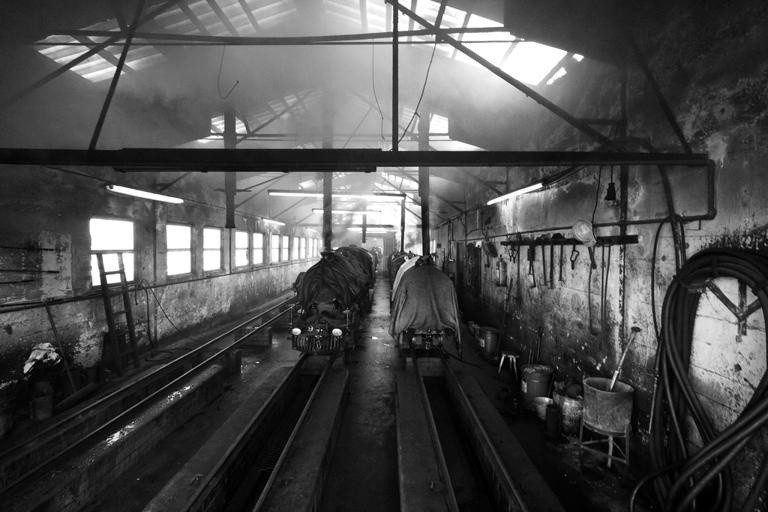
[96,252,139,376]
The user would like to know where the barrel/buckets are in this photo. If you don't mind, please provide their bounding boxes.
[582,376,633,435]
[522,367,552,408]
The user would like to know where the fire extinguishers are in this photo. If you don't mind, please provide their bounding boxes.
[495,254,506,287]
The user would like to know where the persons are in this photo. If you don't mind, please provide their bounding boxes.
[432,243,444,270]
